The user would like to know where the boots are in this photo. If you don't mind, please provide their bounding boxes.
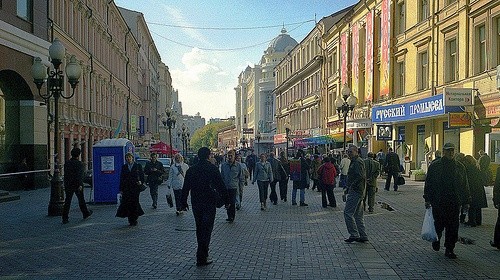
[460,215,466,225]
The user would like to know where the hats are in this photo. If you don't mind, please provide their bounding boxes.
[443,143,456,149]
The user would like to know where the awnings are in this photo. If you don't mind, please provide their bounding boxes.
[371,87,478,127]
[305,136,336,146]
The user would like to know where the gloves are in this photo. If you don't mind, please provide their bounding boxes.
[182,201,190,212]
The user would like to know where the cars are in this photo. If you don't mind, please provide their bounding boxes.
[136,159,150,170]
[83,168,92,185]
[158,158,172,168]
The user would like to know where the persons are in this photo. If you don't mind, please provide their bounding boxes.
[424,143,500,259]
[167,153,190,214]
[342,145,372,244]
[18,159,38,191]
[177,146,233,268]
[61,146,94,224]
[114,153,146,227]
[384,147,402,191]
[144,153,163,208]
[188,144,385,213]
[220,150,244,224]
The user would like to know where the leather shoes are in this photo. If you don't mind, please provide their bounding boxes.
[197,260,213,267]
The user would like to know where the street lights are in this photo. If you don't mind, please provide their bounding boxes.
[161,106,177,157]
[177,123,191,156]
[256,130,262,155]
[334,83,358,151]
[30,38,82,215]
[284,122,291,161]
[240,134,248,149]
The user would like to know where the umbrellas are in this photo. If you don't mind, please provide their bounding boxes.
[148,141,179,156]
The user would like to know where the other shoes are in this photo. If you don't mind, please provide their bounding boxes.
[465,221,482,227]
[62,221,69,224]
[445,251,456,259]
[84,210,93,220]
[129,221,138,226]
[368,210,374,214]
[432,242,440,252]
[344,236,369,243]
[261,206,264,210]
[293,203,308,206]
[490,241,500,249]
[176,211,183,216]
[225,217,234,222]
[152,204,158,208]
[385,187,389,191]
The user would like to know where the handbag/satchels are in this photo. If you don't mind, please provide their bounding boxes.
[397,173,405,185]
[136,163,147,193]
[117,194,122,210]
[166,187,174,209]
[421,207,439,243]
[209,187,225,208]
